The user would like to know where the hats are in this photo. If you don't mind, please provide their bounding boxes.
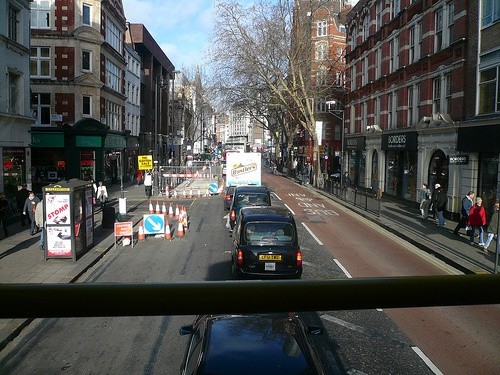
[493,203,499,208]
[435,184,441,189]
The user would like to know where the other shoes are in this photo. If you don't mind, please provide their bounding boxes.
[483,247,488,253]
[434,218,446,227]
[478,242,485,246]
[453,231,462,236]
[470,241,474,244]
[474,234,479,238]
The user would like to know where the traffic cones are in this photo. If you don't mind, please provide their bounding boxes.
[197,189,202,198]
[162,202,168,216]
[155,200,161,214]
[169,202,175,217]
[165,219,174,241]
[205,188,213,200]
[137,220,146,241]
[175,203,180,220]
[177,206,190,236]
[148,201,155,215]
[181,186,188,199]
[173,188,180,199]
[165,188,171,199]
[187,186,194,199]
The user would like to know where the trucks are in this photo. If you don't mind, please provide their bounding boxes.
[221,149,263,188]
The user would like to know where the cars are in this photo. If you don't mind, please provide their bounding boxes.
[180,312,326,375]
[228,185,272,237]
[223,183,257,212]
[230,206,303,280]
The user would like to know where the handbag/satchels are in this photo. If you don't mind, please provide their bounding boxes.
[93,199,96,204]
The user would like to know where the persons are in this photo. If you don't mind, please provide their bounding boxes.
[91,179,108,210]
[483,202,500,255]
[388,175,398,190]
[372,177,379,196]
[131,168,155,197]
[277,229,284,236]
[17,182,44,250]
[247,224,255,236]
[343,172,351,196]
[453,191,487,245]
[293,157,312,178]
[420,184,447,227]
[0,192,9,236]
